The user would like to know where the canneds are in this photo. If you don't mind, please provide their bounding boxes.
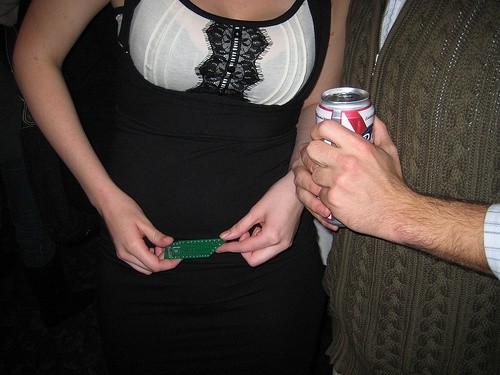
[315,86,376,228]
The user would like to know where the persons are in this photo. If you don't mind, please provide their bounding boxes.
[12,0,352,375]
[291,0,499,375]
